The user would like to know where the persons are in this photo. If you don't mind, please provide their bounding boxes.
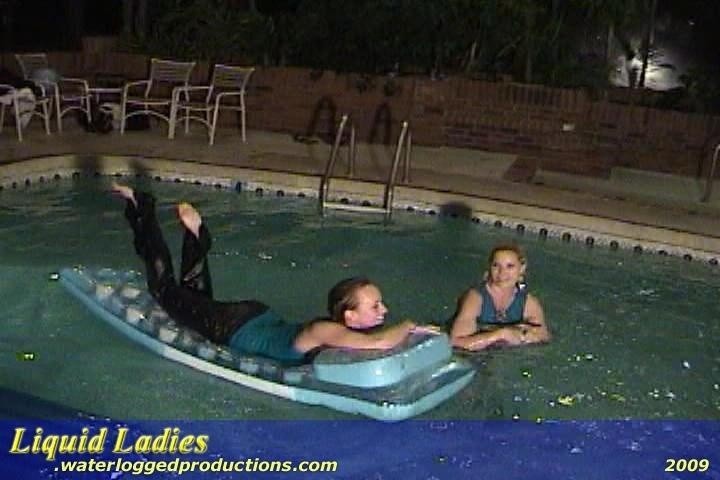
[450,244,551,354]
[108,176,440,367]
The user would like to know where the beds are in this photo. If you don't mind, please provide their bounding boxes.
[88,84,122,105]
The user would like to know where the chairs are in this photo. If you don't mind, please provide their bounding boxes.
[14,53,93,135]
[168,64,256,146]
[119,57,197,139]
[0,66,50,142]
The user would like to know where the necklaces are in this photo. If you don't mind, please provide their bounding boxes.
[495,296,514,319]
